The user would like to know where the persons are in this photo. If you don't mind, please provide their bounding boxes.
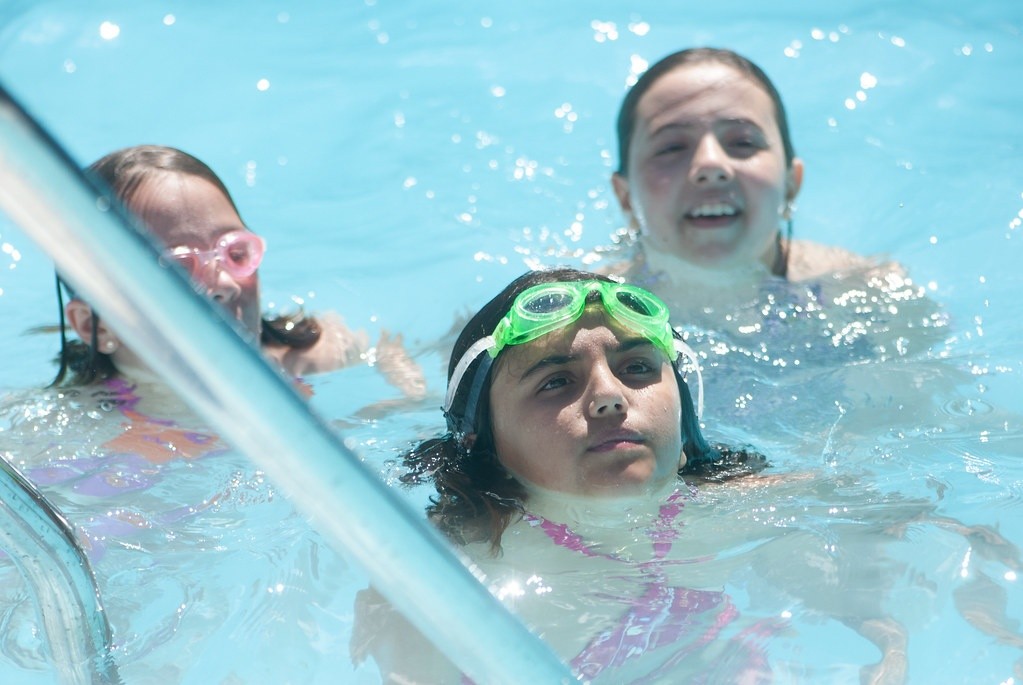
[440,268,709,496]
[611,49,850,283]
[54,145,323,378]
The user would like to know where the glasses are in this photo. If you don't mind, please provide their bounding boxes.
[485,279,679,362]
[161,228,266,306]
